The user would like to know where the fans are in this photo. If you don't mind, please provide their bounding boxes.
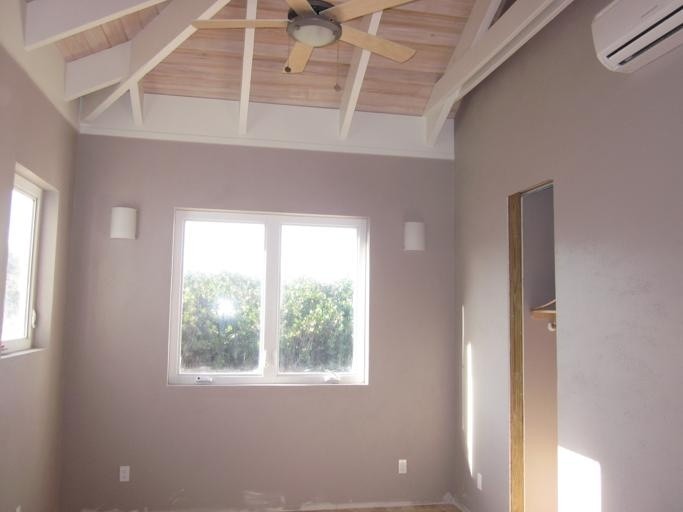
[196,0,419,93]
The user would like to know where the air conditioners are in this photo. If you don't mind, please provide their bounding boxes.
[590,0,683,74]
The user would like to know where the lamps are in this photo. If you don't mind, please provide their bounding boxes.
[110,205,137,241]
[402,221,426,256]
